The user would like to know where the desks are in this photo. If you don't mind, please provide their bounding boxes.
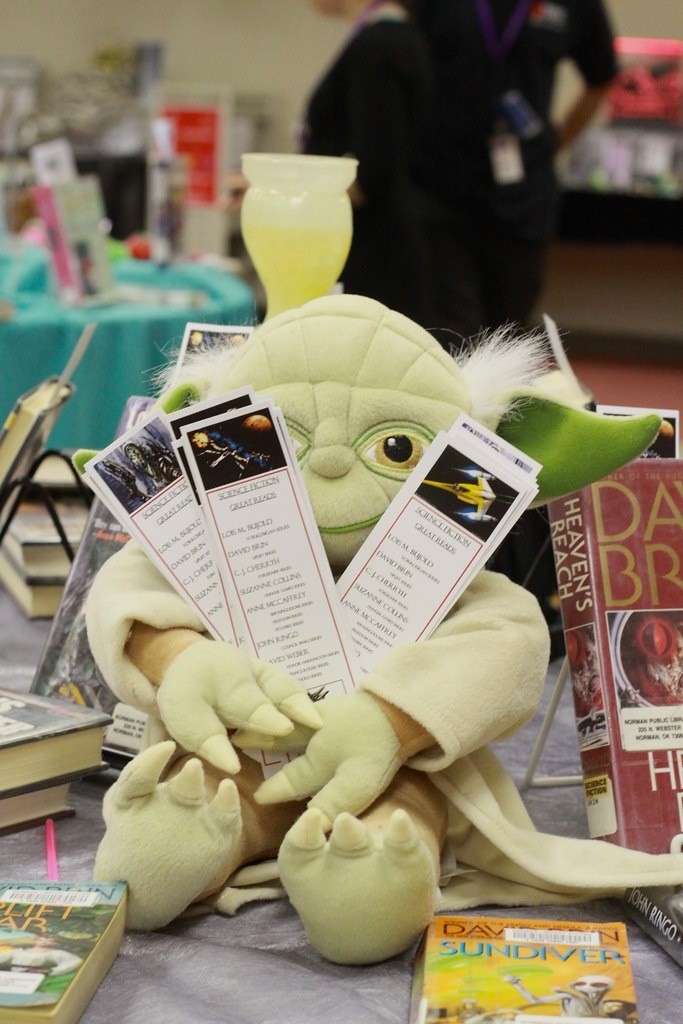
[0,236,257,452]
[0,584,683,1024]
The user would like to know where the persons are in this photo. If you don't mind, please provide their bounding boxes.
[298,0,617,359]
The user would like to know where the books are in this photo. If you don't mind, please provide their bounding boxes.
[0,881,128,1024]
[0,687,114,839]
[547,460,683,853]
[409,917,640,1024]
[0,378,92,622]
[30,396,159,771]
[623,884,683,968]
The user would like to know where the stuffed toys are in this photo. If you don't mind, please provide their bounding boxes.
[70,291,665,965]
